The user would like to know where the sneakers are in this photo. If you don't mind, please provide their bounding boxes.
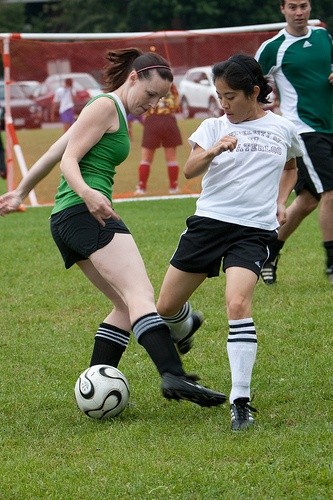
[261,250,283,289]
[229,397,255,433]
[167,185,181,194]
[178,310,206,355]
[131,184,147,196]
[160,372,227,408]
[324,264,333,276]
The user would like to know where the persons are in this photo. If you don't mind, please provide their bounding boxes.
[128,83,183,195]
[254,0,333,286]
[0,48,229,408]
[51,78,77,132]
[155,53,305,433]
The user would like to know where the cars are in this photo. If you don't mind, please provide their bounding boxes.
[0,81,42,130]
[29,73,105,121]
[177,66,224,120]
[16,81,42,99]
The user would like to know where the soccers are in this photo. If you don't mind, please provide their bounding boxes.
[72,362,132,422]
